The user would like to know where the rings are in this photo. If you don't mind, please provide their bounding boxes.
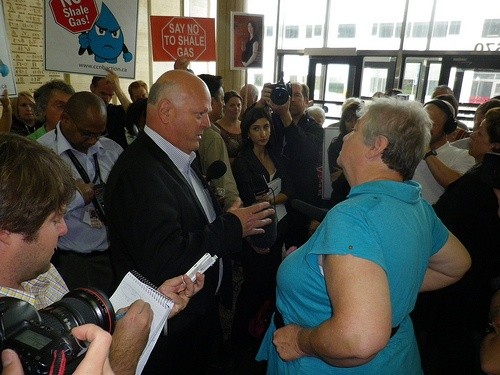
[184,289,192,298]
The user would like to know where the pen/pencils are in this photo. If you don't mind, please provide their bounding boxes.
[115,311,127,320]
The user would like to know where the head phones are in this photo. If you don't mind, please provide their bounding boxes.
[438,97,458,135]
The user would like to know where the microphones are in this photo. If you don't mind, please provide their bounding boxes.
[205,159,228,184]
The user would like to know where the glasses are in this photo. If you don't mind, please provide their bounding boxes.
[74,122,108,140]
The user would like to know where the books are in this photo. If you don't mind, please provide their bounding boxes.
[109,270,175,375]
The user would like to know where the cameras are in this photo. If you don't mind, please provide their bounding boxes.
[0,286,117,374]
[266,77,292,105]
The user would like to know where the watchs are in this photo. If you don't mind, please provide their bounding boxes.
[423,148,436,160]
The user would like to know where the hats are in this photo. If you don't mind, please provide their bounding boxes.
[341,97,361,113]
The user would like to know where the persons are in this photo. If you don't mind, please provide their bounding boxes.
[268,97,472,375]
[0,134,205,375]
[2,323,115,375]
[0,57,498,375]
[103,71,275,375]
[240,21,260,68]
